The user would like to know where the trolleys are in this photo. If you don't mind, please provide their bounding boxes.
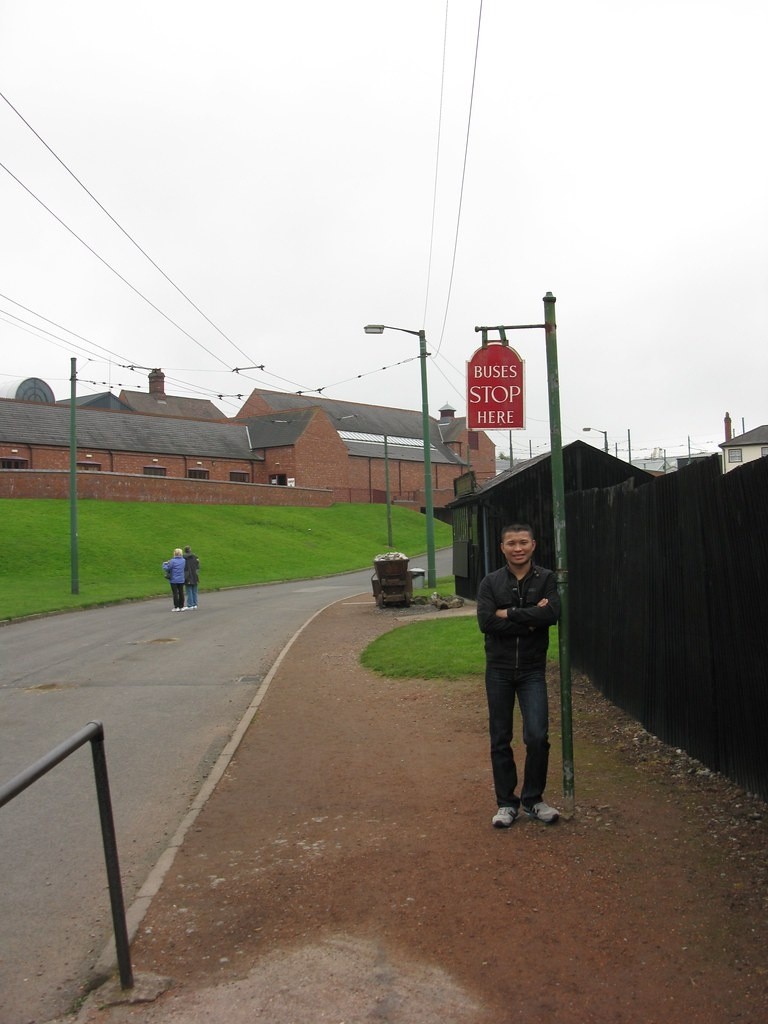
[370,560,413,610]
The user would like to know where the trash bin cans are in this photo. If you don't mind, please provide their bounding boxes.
[371,551,410,579]
[409,568,424,589]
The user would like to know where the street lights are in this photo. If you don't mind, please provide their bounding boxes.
[364,325,437,590]
[583,427,609,454]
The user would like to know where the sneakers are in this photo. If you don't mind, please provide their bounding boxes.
[524,802,560,824]
[492,806,517,827]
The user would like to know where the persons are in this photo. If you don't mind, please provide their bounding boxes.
[163,546,200,612]
[477,520,561,827]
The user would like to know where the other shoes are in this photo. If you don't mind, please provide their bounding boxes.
[183,606,197,609]
[172,607,186,611]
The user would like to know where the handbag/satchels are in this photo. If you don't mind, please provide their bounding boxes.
[164,569,171,579]
